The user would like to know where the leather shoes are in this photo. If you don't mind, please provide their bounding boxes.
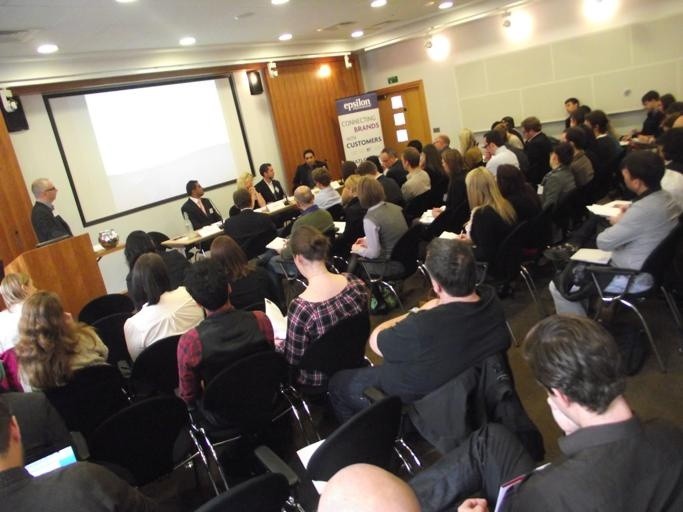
[370,302,389,316]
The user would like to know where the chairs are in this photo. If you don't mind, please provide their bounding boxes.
[0,144,683,511]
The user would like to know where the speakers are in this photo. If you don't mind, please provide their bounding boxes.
[0,95,29,133]
[247,70,263,95]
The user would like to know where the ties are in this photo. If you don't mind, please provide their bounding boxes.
[198,199,207,216]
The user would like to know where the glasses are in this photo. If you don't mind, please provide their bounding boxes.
[43,186,56,193]
[482,143,490,150]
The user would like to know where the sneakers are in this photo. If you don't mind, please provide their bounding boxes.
[621,320,648,376]
[498,283,514,301]
